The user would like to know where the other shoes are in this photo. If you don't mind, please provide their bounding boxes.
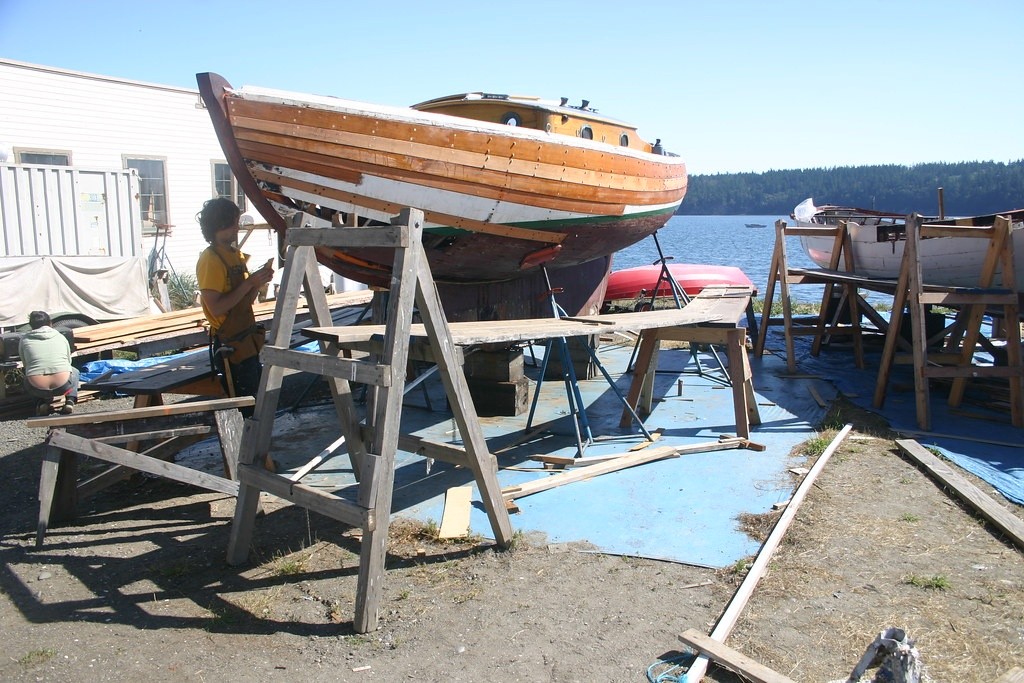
[36,401,49,416]
[62,400,74,413]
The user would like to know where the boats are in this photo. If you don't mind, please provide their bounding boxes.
[224,86,688,278]
[745,223,767,228]
[601,264,760,302]
[791,187,1024,292]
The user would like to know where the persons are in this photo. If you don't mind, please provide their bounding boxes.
[18,309,79,416]
[193,198,275,419]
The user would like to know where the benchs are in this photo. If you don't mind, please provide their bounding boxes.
[26,393,259,546]
[296,283,763,453]
[753,211,1023,432]
[81,302,374,455]
